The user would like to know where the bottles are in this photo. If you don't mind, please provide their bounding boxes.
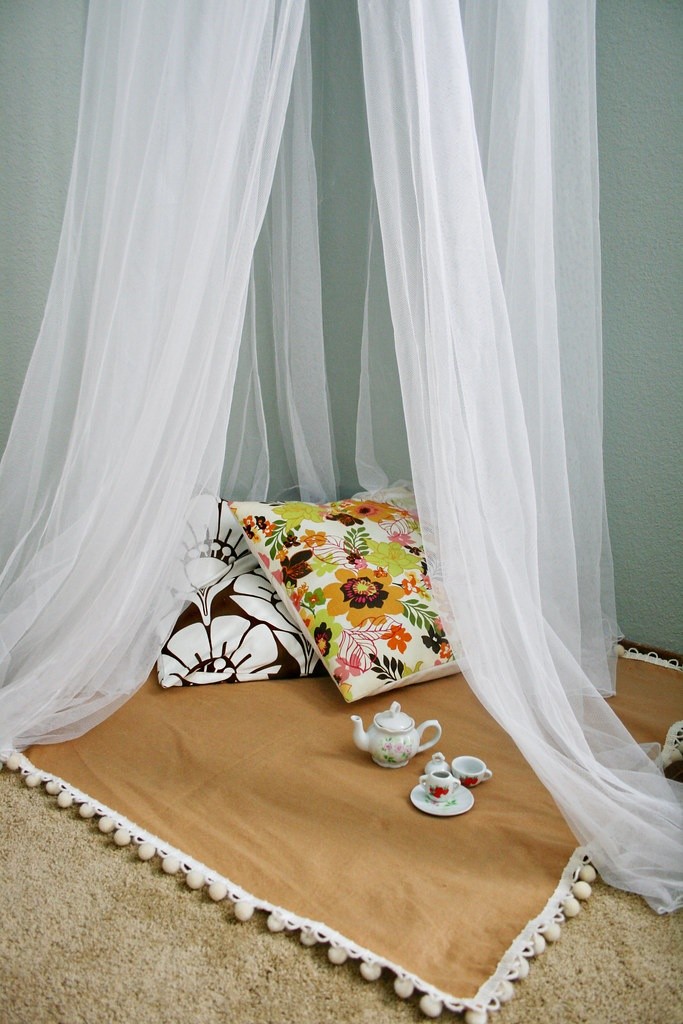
[419,770,461,801]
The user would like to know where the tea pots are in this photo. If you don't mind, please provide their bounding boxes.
[350,701,442,768]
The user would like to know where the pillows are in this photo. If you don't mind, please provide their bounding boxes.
[155,492,331,689]
[228,487,463,702]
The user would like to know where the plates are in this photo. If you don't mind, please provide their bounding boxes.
[410,783,474,816]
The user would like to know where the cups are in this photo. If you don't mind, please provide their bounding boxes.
[451,756,492,788]
